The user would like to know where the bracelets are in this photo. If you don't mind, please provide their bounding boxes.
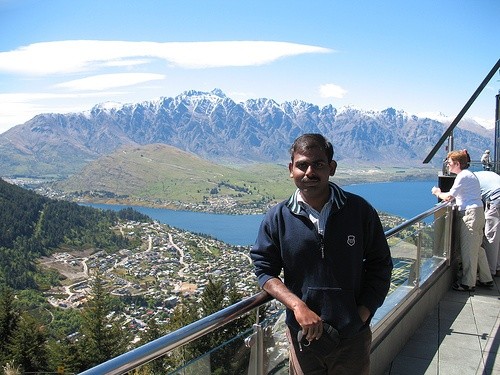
[437,193,441,198]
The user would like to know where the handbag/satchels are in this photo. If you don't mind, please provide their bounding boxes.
[301,321,340,358]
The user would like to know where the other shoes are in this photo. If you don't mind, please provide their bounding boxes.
[454,283,476,291]
[476,280,494,288]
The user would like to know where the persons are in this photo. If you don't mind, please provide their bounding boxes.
[431,148,500,292]
[250,134,393,375]
